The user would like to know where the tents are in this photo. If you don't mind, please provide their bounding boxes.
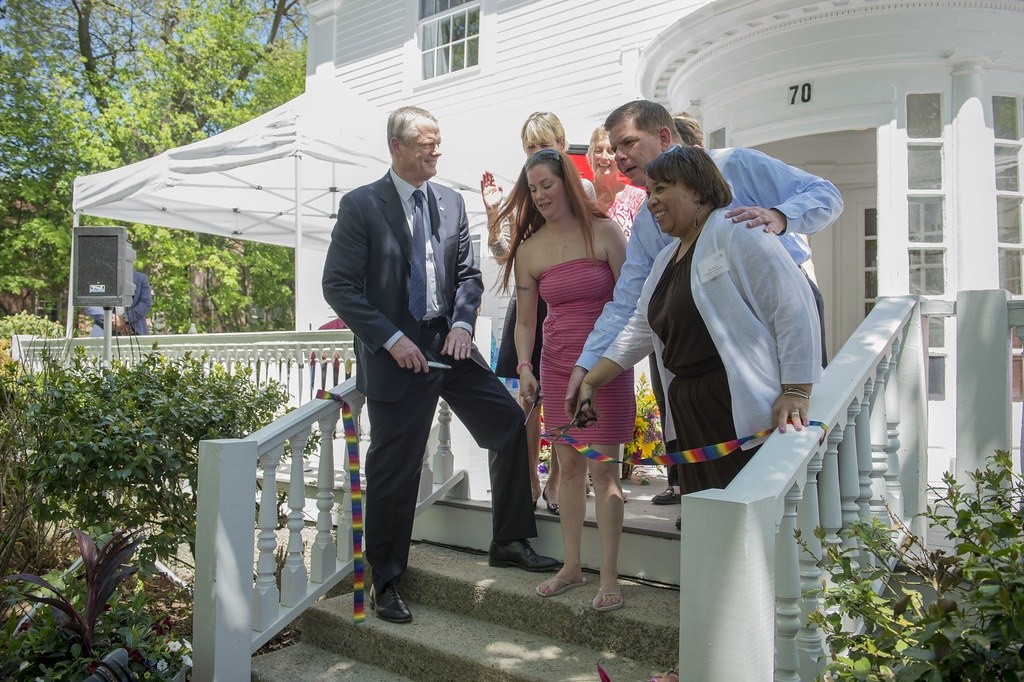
[65,77,527,338]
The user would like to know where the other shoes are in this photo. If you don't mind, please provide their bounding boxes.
[622,492,628,502]
[543,486,560,515]
[651,486,681,504]
[676,515,681,529]
[531,487,541,509]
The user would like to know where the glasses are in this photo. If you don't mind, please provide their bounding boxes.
[528,149,562,161]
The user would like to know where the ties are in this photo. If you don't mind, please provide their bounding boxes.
[408,190,427,322]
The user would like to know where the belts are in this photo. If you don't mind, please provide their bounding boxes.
[421,317,445,328]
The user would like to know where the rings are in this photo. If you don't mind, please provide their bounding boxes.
[524,395,530,401]
[461,343,466,347]
[792,412,800,416]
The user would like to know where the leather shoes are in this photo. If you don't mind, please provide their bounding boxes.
[369,583,413,623]
[489,538,560,571]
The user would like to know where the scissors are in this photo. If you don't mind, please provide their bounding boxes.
[546,398,598,447]
[423,353,453,376]
[523,385,543,426]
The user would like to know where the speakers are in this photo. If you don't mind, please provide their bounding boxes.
[73,226,137,307]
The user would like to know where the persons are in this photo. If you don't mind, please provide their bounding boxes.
[323,106,563,623]
[481,111,705,612]
[566,100,844,529]
[81,267,152,338]
[574,147,822,682]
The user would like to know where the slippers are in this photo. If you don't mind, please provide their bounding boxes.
[593,588,624,612]
[536,572,587,596]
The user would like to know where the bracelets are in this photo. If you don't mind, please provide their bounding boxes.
[784,387,811,400]
[517,361,533,374]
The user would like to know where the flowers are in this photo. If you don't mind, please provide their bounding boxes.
[622,371,666,475]
[537,406,551,476]
